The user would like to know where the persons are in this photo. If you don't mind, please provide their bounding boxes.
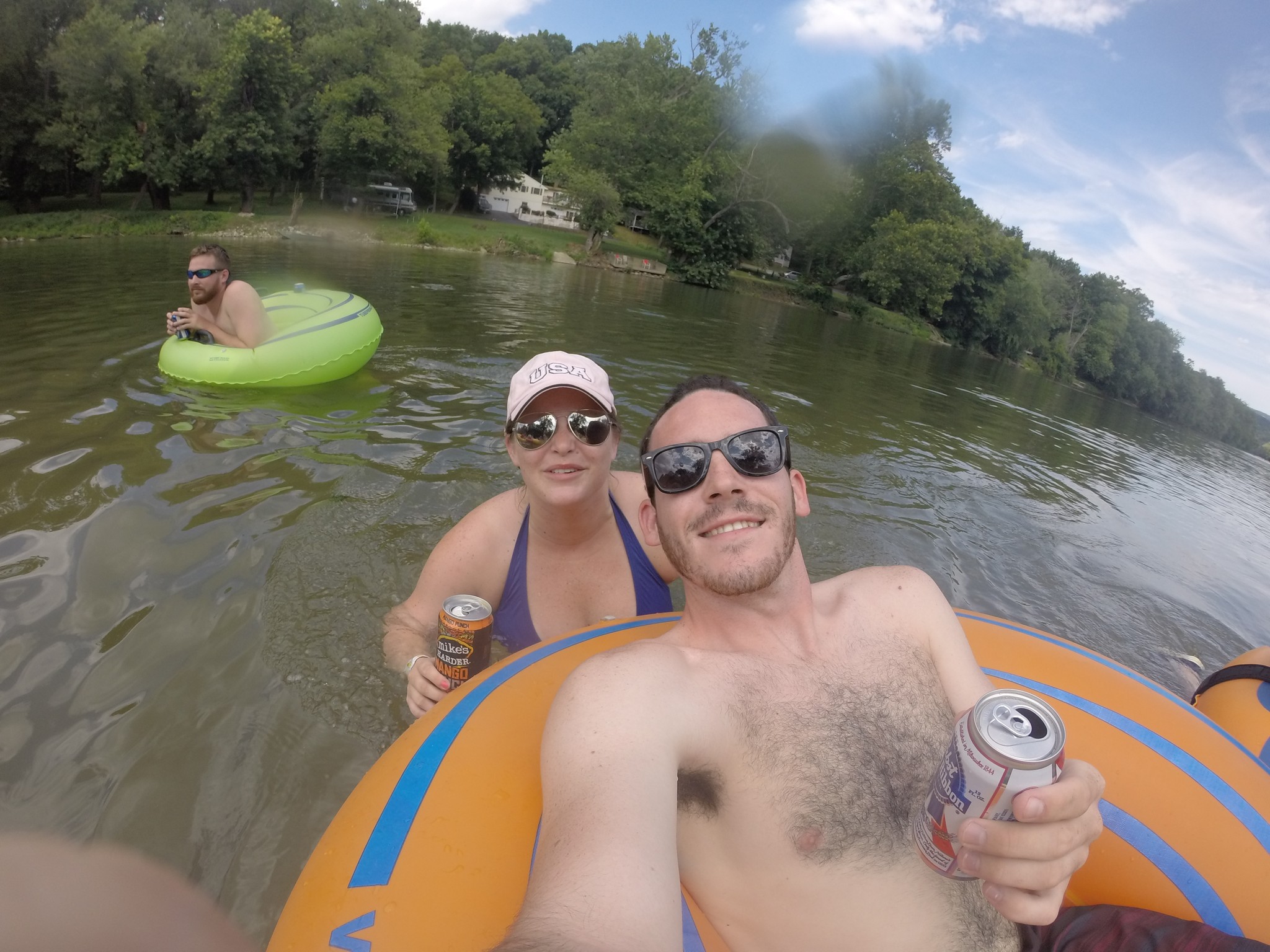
[166,243,275,348]
[382,350,679,718]
[489,372,1270,952]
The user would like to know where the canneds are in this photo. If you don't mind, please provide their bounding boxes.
[171,315,191,341]
[294,283,305,293]
[434,594,494,691]
[911,688,1063,880]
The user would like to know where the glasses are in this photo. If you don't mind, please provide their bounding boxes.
[187,268,225,280]
[506,408,616,451]
[640,426,792,499]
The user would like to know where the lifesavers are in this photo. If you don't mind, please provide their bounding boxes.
[264,603,1270,952]
[156,281,385,387]
[1189,644,1270,771]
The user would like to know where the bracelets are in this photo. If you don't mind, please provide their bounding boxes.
[402,655,429,675]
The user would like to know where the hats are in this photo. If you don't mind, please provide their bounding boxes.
[506,350,617,427]
